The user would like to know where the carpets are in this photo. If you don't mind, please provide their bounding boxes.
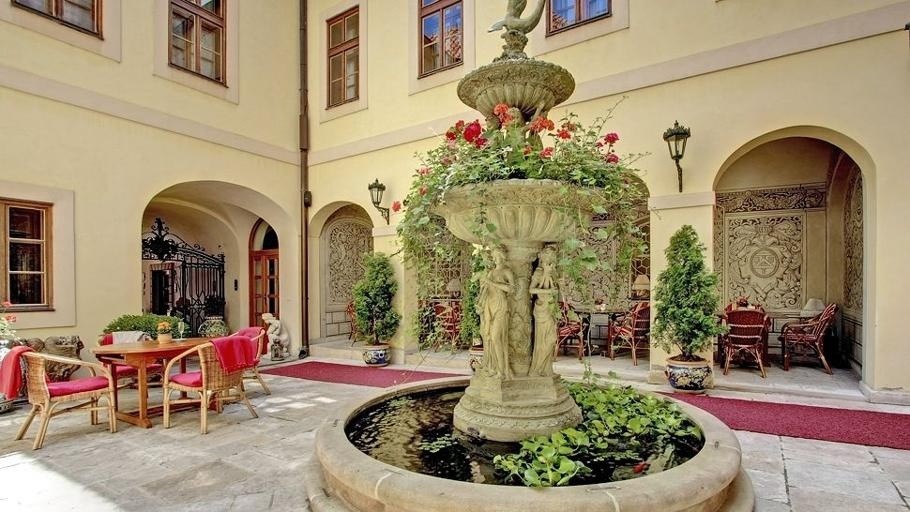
[258,361,910,452]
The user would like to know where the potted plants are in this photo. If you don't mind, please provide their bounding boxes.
[651,224,726,396]
[351,251,401,367]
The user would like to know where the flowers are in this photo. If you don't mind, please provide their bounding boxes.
[391,101,619,213]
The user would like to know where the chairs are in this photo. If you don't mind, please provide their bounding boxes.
[554,301,649,367]
[224,327,271,397]
[99,331,163,410]
[0,345,117,450]
[163,335,258,435]
[719,300,836,378]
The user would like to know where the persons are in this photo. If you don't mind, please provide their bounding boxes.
[479,247,514,381]
[528,247,560,377]
[261,313,290,354]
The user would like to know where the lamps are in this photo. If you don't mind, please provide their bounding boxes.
[663,118,690,193]
[368,178,389,226]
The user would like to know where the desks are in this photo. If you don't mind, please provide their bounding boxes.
[92,336,214,430]
